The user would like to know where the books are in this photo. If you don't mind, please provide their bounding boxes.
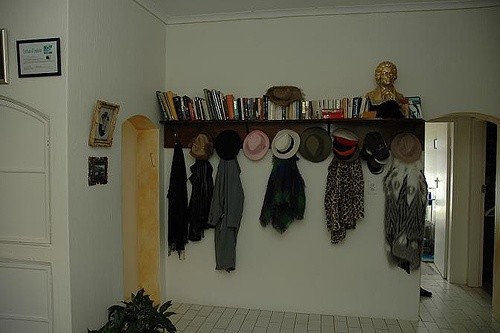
[399,96,424,119]
[155,89,370,120]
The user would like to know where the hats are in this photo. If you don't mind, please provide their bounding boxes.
[332,128,360,163]
[299,127,332,162]
[376,100,403,119]
[243,130,270,160]
[271,129,300,160]
[391,131,421,162]
[214,130,241,160]
[267,86,302,106]
[101,112,109,121]
[190,134,213,161]
[360,132,390,174]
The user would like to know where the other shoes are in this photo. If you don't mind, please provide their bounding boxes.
[420,287,432,297]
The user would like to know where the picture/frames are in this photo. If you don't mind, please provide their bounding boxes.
[88,99,120,146]
[0,28,9,84]
[88,157,108,186]
[16,38,61,78]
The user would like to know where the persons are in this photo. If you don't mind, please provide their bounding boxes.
[95,168,101,176]
[99,112,109,136]
[366,61,405,106]
